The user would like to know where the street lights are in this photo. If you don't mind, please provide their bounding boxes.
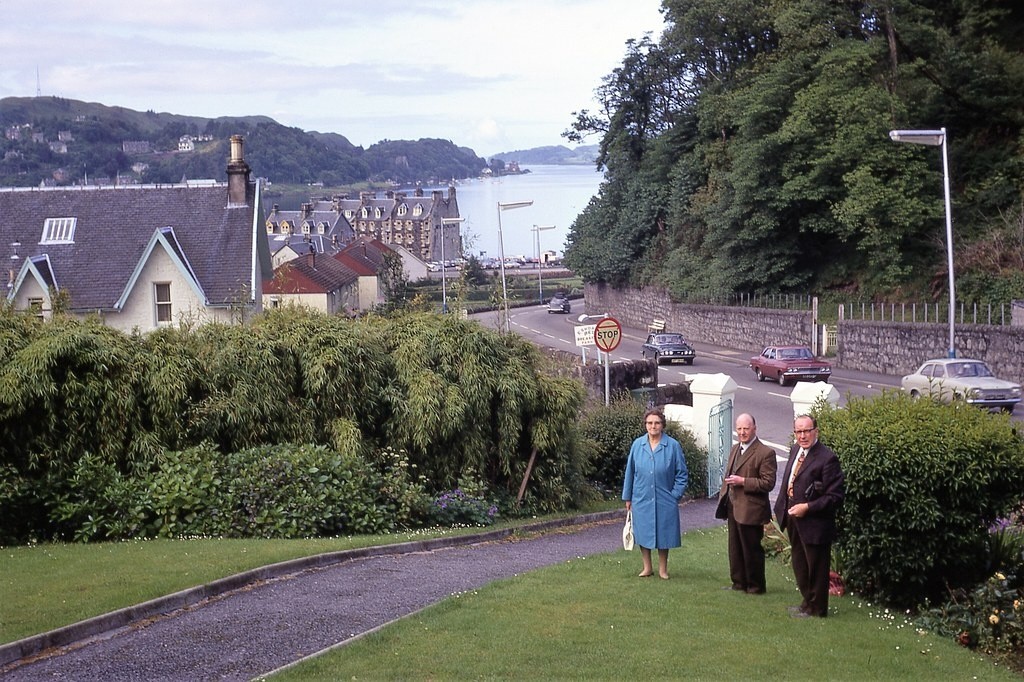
[496,200,534,338]
[440,217,465,316]
[578,313,614,409]
[890,126,957,358]
[536,225,556,305]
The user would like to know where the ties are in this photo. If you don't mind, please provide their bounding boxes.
[787,452,805,499]
[736,446,743,461]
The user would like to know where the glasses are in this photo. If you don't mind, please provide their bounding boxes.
[793,427,815,432]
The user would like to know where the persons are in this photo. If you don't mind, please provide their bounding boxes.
[942,366,959,378]
[773,415,844,618]
[622,411,688,580]
[778,351,783,359]
[714,413,777,594]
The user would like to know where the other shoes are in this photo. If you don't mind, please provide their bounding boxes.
[639,570,654,576]
[797,610,826,618]
[722,582,746,590]
[747,587,766,594]
[786,600,808,611]
[659,574,669,580]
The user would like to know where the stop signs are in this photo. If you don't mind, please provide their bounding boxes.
[594,317,621,353]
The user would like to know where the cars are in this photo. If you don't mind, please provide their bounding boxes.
[547,297,571,315]
[750,346,832,386]
[482,256,530,270]
[641,333,695,362]
[901,358,1024,414]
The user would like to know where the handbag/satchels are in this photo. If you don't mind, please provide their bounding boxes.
[622,510,635,550]
